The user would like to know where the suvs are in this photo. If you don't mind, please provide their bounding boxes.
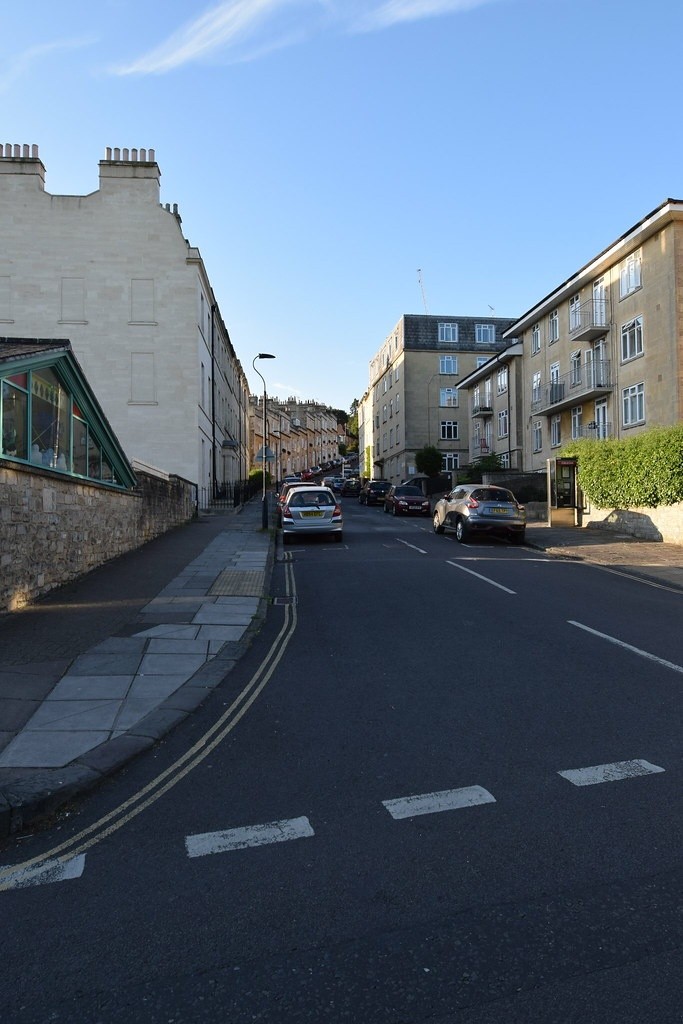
[274,482,319,520]
[348,452,357,461]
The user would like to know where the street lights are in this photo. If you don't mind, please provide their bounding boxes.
[253,354,275,528]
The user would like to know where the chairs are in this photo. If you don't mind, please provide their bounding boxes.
[296,497,303,502]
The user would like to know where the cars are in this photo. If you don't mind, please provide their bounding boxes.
[334,458,341,467]
[295,472,304,481]
[328,461,337,469]
[284,474,297,478]
[319,462,332,472]
[339,480,362,498]
[320,477,335,488]
[311,466,324,476]
[278,487,345,537]
[355,454,359,459]
[381,485,431,517]
[341,456,351,464]
[281,478,302,485]
[358,480,393,506]
[331,478,346,491]
[433,483,527,542]
[302,468,314,479]
